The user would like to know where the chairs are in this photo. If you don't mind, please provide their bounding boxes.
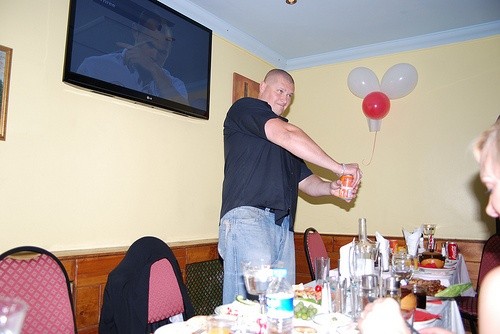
[95,233,195,334]
[303,227,332,281]
[0,244,78,334]
[452,233,500,334]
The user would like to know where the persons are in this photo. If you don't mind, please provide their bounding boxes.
[218,68,361,305]
[76,10,188,104]
[357,114,500,334]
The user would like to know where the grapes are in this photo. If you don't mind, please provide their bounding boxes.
[294,301,318,320]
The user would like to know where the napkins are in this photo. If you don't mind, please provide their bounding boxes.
[339,224,424,276]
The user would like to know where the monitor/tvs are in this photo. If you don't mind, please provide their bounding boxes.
[62,0,212,120]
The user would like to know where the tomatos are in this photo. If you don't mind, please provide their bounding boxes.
[315,286,321,292]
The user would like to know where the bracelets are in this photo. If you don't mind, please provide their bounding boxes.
[335,164,346,176]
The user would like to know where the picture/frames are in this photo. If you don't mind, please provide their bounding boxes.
[0,42,14,149]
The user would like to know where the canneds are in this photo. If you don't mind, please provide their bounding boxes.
[339,174,355,200]
[448,242,458,260]
[390,240,398,255]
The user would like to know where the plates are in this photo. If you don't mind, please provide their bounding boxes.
[152,260,457,333]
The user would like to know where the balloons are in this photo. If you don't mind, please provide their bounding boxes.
[362,91,390,119]
[347,66,380,98]
[381,63,418,100]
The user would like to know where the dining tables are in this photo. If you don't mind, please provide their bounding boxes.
[158,262,500,334]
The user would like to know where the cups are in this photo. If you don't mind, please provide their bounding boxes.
[243,225,436,334]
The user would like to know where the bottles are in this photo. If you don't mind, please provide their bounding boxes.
[266,269,293,334]
[354,219,374,277]
[319,281,331,314]
[441,243,447,257]
